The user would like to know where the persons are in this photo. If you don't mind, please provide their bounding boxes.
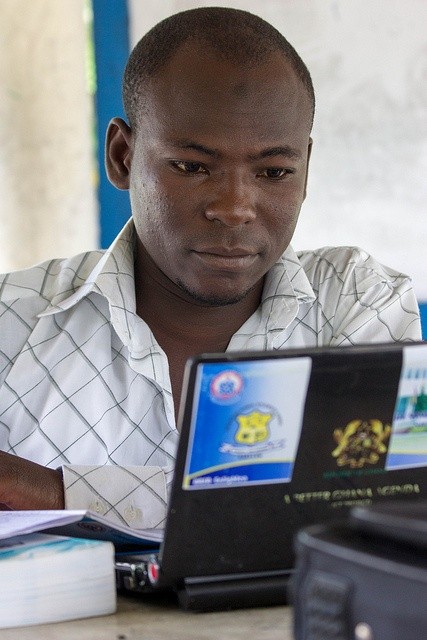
[0,6,423,530]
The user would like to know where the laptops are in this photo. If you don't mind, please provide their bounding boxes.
[115,342,427,612]
